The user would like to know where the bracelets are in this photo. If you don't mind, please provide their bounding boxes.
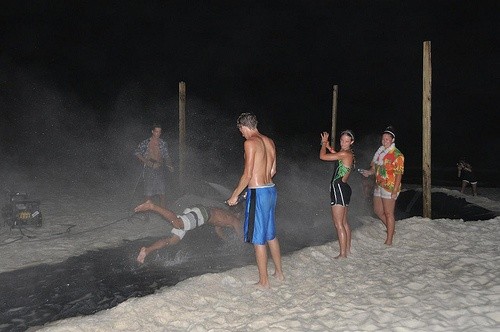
[329,146,333,151]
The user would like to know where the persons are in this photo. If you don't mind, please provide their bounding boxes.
[358,127,404,246]
[225,113,286,287]
[138,124,176,209]
[319,130,357,259]
[134,199,245,264]
[457,160,478,196]
